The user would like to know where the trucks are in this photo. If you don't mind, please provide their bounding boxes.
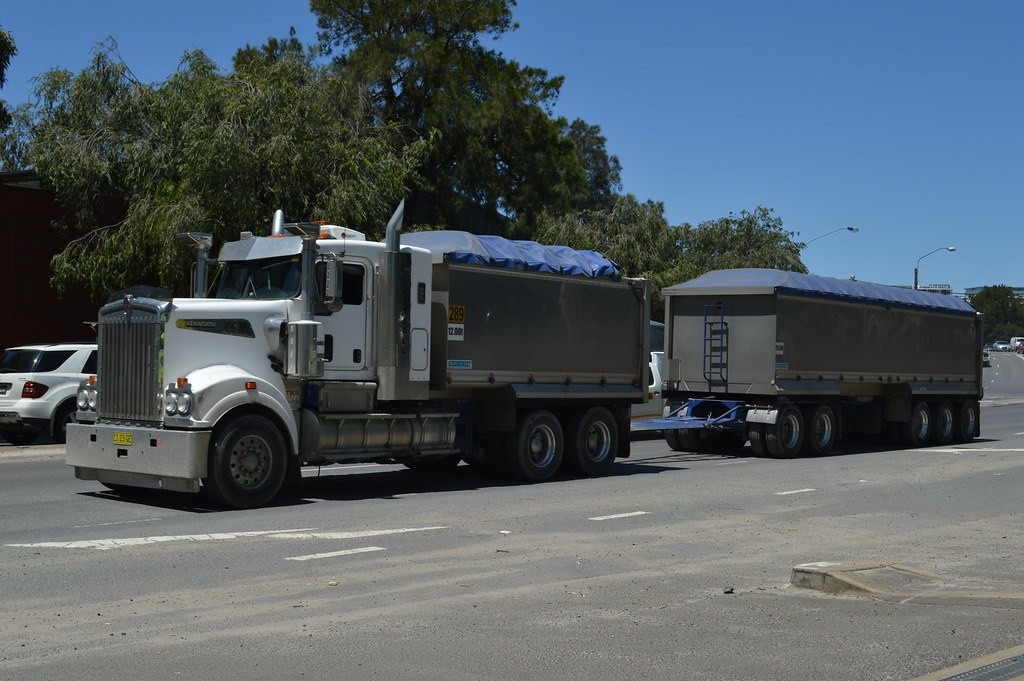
[983,349,991,367]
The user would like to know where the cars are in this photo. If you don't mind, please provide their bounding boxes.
[630,320,665,423]
[985,343,993,351]
[1010,337,1024,351]
[993,341,1012,351]
[1016,341,1024,354]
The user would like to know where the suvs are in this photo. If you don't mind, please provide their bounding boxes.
[0,341,98,446]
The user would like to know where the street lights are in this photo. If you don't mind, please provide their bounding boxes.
[805,227,859,245]
[914,247,957,290]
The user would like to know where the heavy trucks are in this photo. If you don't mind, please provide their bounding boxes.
[65,196,984,511]
